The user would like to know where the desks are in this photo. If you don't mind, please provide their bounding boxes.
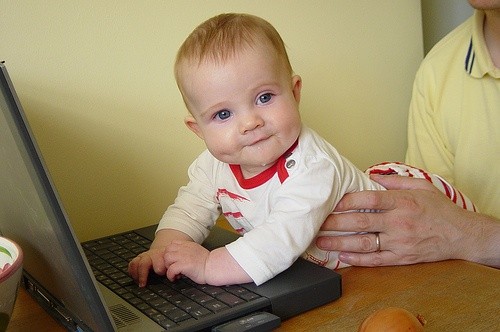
[5,214,500,332]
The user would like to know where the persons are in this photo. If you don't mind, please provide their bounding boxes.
[128,12,479,289]
[316,0,500,270]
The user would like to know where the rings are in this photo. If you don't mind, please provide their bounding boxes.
[375,231,381,253]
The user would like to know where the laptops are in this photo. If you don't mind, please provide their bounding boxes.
[0,63,343,332]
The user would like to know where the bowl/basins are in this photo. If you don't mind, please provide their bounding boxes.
[0,235,23,331]
